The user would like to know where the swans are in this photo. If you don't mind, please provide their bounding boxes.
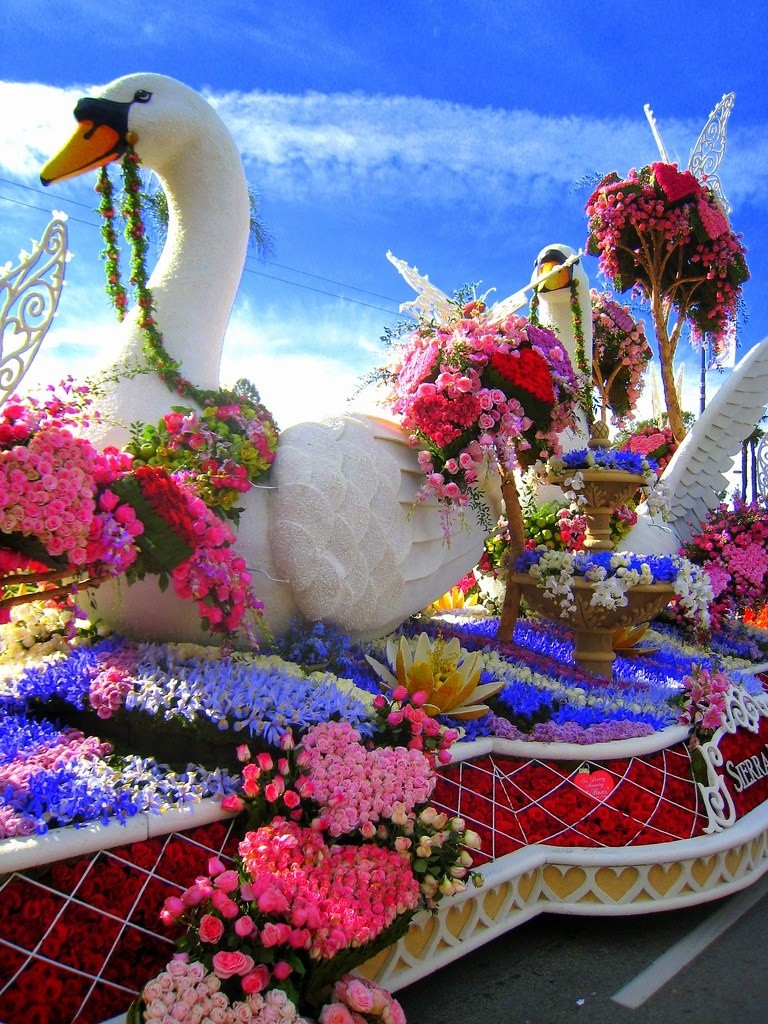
[39,70,768,652]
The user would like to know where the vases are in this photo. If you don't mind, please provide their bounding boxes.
[0,682,766,1024]
[548,421,648,550]
[512,570,677,679]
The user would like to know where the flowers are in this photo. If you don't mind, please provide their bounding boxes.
[0,127,767,1024]
